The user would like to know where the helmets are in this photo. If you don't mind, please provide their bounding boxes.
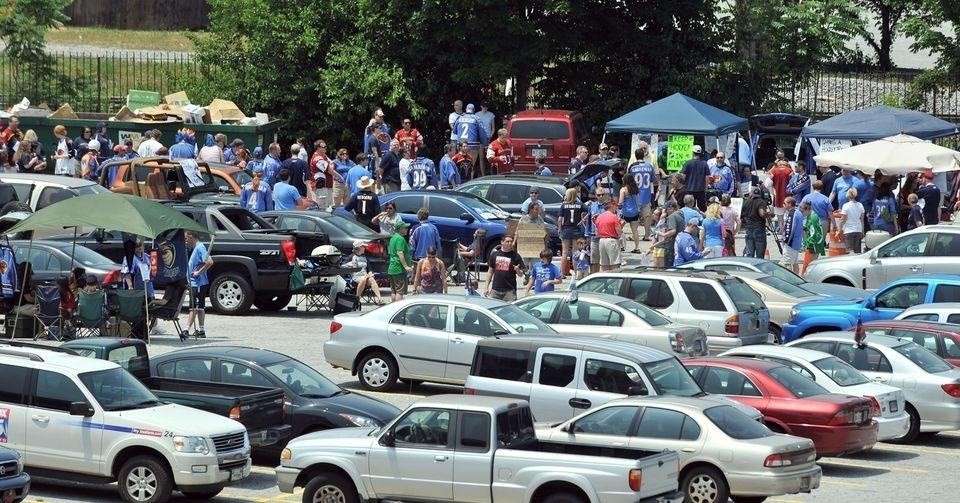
[88,139,101,152]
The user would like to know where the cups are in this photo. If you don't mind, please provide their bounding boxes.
[579,250,586,260]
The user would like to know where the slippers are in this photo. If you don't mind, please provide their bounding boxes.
[626,236,634,241]
[642,236,649,241]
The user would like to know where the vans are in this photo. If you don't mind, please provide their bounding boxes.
[501,110,591,172]
[747,113,814,179]
[0,173,116,239]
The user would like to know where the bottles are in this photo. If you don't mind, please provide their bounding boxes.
[407,264,415,279]
[352,255,358,268]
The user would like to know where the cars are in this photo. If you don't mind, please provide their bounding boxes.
[1,239,141,326]
[1,444,31,503]
[632,257,960,368]
[475,291,708,357]
[150,345,416,456]
[630,355,878,461]
[246,211,391,291]
[767,330,960,445]
[801,224,959,291]
[538,394,822,502]
[330,189,561,269]
[449,175,584,220]
[323,293,598,393]
[687,344,910,441]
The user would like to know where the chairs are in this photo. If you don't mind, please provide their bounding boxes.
[74,288,106,340]
[152,168,173,200]
[146,172,158,200]
[149,285,187,342]
[34,285,63,340]
[348,254,379,305]
[451,234,485,281]
[115,289,148,342]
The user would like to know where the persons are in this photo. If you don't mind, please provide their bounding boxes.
[1,99,960,340]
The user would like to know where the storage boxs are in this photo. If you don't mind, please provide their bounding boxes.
[5,316,38,337]
[116,88,245,125]
[517,223,548,259]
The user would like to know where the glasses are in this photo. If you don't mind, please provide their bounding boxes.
[126,143,132,146]
[483,105,487,106]
[597,193,604,196]
[529,191,537,194]
[321,122,411,154]
[603,148,608,149]
[717,156,723,159]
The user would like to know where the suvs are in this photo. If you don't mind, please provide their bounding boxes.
[108,157,256,205]
[0,338,251,503]
[566,267,769,354]
[463,335,766,425]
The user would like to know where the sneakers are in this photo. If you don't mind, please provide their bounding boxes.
[182,330,189,337]
[523,271,530,285]
[621,246,625,251]
[193,330,206,338]
[631,249,641,254]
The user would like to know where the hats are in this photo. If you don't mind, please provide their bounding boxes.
[356,175,375,188]
[96,121,168,154]
[662,186,761,229]
[375,109,385,116]
[599,142,608,149]
[352,240,368,247]
[480,98,489,105]
[229,137,264,173]
[692,144,702,153]
[395,220,411,231]
[924,169,934,179]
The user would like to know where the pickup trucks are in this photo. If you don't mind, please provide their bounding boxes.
[38,199,331,314]
[56,336,293,447]
[275,393,680,503]
